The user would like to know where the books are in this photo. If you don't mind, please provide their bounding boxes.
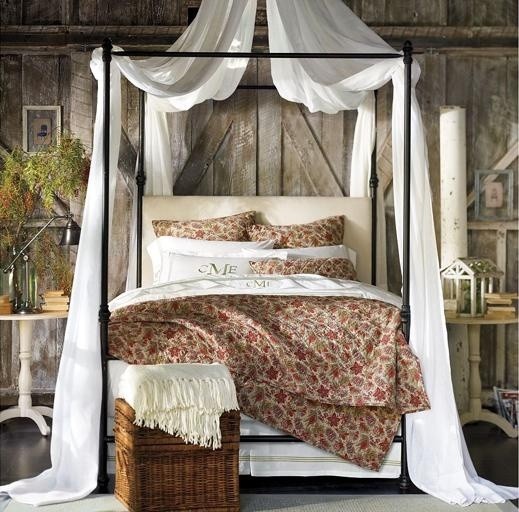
[493,387,519,430]
[483,292,518,320]
[39,289,69,312]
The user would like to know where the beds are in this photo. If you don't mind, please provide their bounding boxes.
[92,35,420,501]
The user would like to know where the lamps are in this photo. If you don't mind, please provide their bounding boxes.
[2,215,81,315]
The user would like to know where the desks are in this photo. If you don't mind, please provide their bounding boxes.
[0,312,69,438]
[438,309,519,439]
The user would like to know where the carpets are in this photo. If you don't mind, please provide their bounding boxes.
[0,491,519,512]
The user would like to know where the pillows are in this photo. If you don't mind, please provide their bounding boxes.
[144,209,361,288]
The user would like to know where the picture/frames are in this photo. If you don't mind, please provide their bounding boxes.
[19,102,64,160]
[474,169,514,223]
